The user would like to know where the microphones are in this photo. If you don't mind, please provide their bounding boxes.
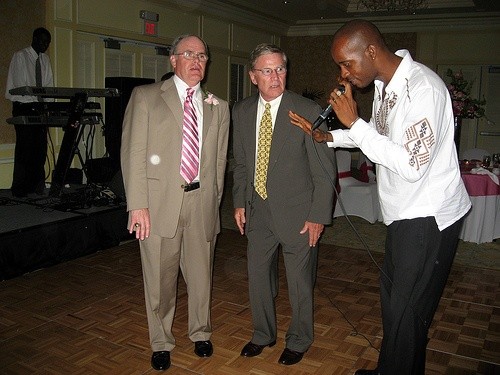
[311,82,355,130]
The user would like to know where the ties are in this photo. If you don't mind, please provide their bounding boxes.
[179,88,199,184]
[35,57,44,103]
[254,103,273,200]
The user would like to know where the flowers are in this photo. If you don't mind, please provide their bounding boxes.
[203,90,219,111]
[445,67,496,126]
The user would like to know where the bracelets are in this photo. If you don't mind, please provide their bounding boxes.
[349,117,360,128]
[322,130,328,144]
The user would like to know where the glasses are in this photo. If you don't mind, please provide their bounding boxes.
[253,65,287,76]
[174,50,209,62]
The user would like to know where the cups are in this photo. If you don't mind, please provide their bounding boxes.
[493,153,500,168]
[483,156,490,168]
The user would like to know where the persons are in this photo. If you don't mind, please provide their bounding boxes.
[288,18,472,375]
[120,34,231,370]
[2,27,55,196]
[232,40,339,363]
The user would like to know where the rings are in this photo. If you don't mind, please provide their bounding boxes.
[135,223,139,227]
[336,91,342,97]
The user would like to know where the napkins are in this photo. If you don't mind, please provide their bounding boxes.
[471,167,499,185]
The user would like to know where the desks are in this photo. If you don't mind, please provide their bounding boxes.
[458,160,500,244]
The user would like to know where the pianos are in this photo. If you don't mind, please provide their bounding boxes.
[5,86,122,209]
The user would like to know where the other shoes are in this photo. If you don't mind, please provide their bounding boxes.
[11,191,34,197]
[354,368,379,375]
[35,192,46,195]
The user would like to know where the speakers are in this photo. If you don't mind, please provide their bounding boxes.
[105,76,155,157]
[86,158,122,185]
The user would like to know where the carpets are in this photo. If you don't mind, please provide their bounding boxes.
[220,192,500,269]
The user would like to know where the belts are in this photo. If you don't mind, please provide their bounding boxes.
[183,182,199,192]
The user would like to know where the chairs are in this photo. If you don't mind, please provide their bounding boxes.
[332,150,379,224]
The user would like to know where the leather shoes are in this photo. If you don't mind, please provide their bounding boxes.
[151,350,171,371]
[239,340,276,357]
[194,340,213,358]
[278,346,305,365]
